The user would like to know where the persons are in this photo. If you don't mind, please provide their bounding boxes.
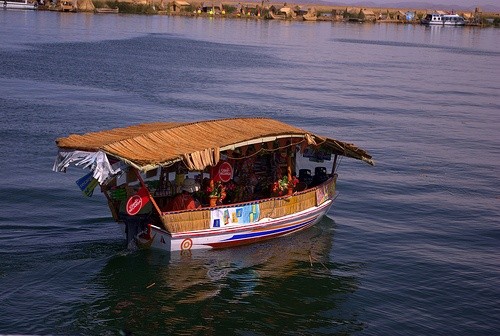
[163,178,202,212]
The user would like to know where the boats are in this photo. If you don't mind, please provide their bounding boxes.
[421,14,466,25]
[55,118,375,253]
